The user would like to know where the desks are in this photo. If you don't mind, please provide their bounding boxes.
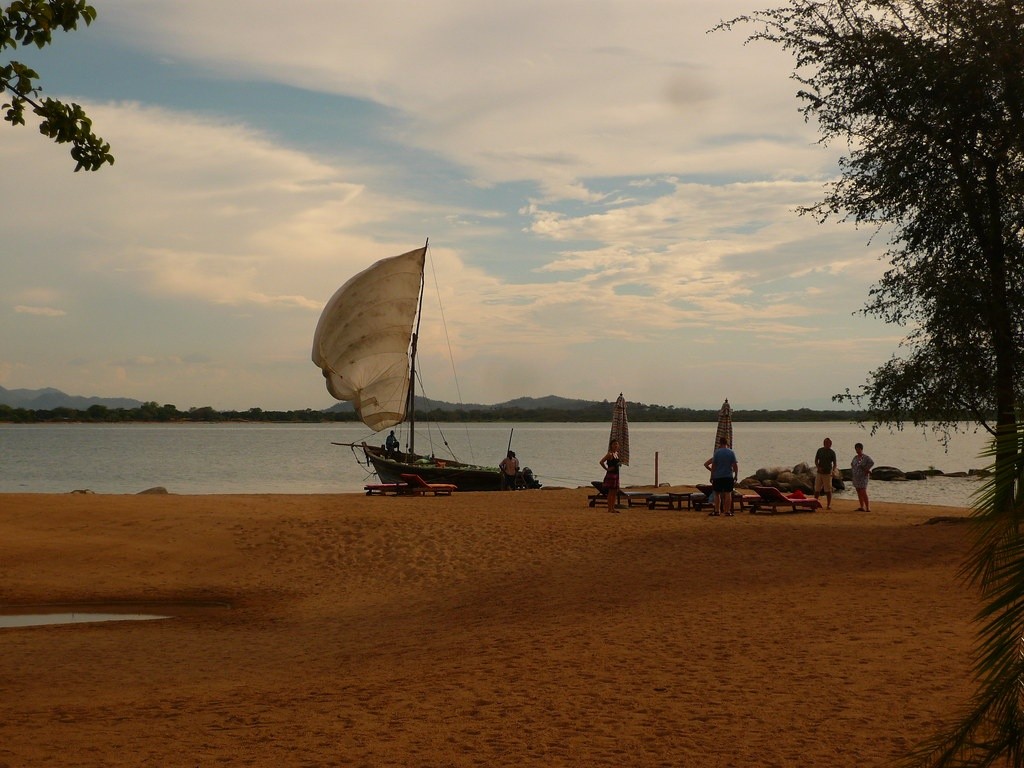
[666,491,693,510]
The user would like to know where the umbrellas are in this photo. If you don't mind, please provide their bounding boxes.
[606,395,630,467]
[714,402,732,452]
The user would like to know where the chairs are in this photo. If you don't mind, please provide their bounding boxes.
[588,479,823,515]
[364,473,458,497]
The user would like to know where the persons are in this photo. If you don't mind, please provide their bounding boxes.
[704,437,738,516]
[386,430,401,453]
[814,438,837,510]
[851,443,874,512]
[499,450,520,478]
[599,439,621,513]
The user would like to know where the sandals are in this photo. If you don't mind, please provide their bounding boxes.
[708,511,720,516]
[725,513,734,516]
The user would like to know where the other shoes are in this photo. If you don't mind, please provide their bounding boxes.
[854,507,871,512]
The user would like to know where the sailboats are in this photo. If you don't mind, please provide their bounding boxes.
[310,236,521,492]
